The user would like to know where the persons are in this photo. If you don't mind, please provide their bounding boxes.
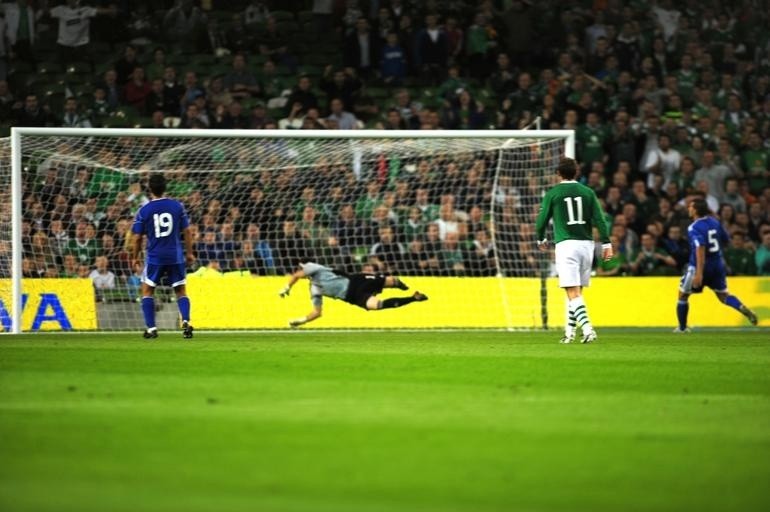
[536,157,613,344]
[672,198,758,335]
[131,175,196,339]
[279,256,429,327]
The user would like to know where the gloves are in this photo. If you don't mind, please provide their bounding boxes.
[289,317,308,329]
[279,285,291,299]
[537,238,551,253]
[600,242,616,264]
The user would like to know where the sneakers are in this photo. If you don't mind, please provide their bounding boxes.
[401,283,409,291]
[748,311,759,327]
[413,290,428,302]
[143,329,158,338]
[672,327,691,333]
[558,332,597,343]
[182,322,193,338]
